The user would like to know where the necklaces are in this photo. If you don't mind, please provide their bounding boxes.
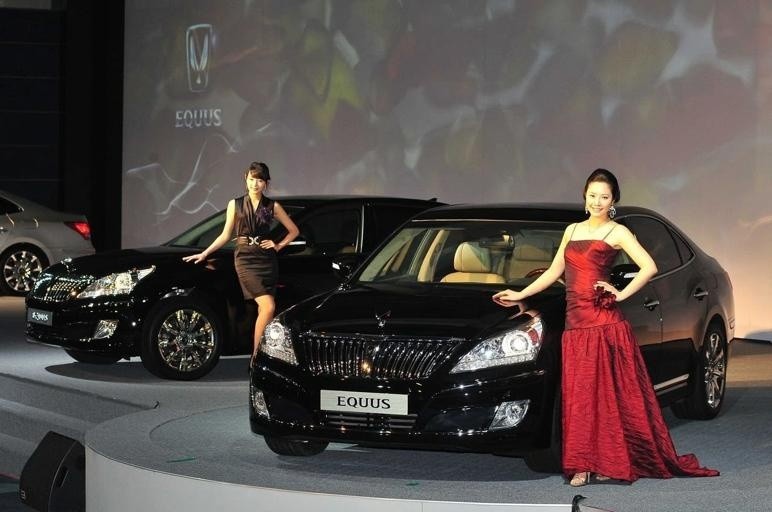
[587,215,610,233]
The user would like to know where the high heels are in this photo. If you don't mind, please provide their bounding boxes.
[570,472,590,486]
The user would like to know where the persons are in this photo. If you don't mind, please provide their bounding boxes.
[182,162,300,381]
[492,167,720,486]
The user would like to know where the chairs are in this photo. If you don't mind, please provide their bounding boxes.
[506,232,563,281]
[437,241,504,283]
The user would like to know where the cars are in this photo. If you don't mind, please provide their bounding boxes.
[22,195,450,382]
[249,202,736,474]
[0,186,98,297]
